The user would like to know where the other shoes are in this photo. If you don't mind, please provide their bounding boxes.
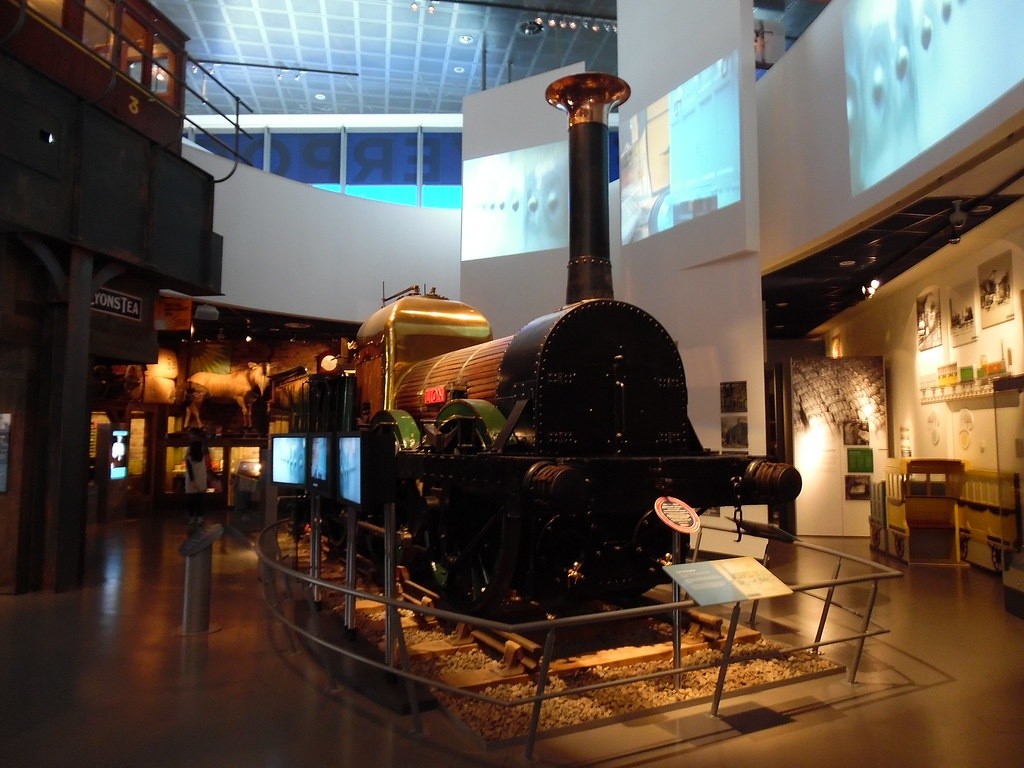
[197,517,203,526]
[189,517,195,525]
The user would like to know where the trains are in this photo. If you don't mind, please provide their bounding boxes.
[266,72,803,620]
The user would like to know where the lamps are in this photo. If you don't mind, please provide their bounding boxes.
[410,0,436,14]
[39,129,52,143]
[535,11,617,32]
[321,354,349,371]
[950,200,967,227]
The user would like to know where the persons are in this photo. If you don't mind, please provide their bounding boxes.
[185,441,206,526]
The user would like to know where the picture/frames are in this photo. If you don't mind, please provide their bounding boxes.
[948,281,979,348]
[978,250,1015,329]
[915,288,942,352]
